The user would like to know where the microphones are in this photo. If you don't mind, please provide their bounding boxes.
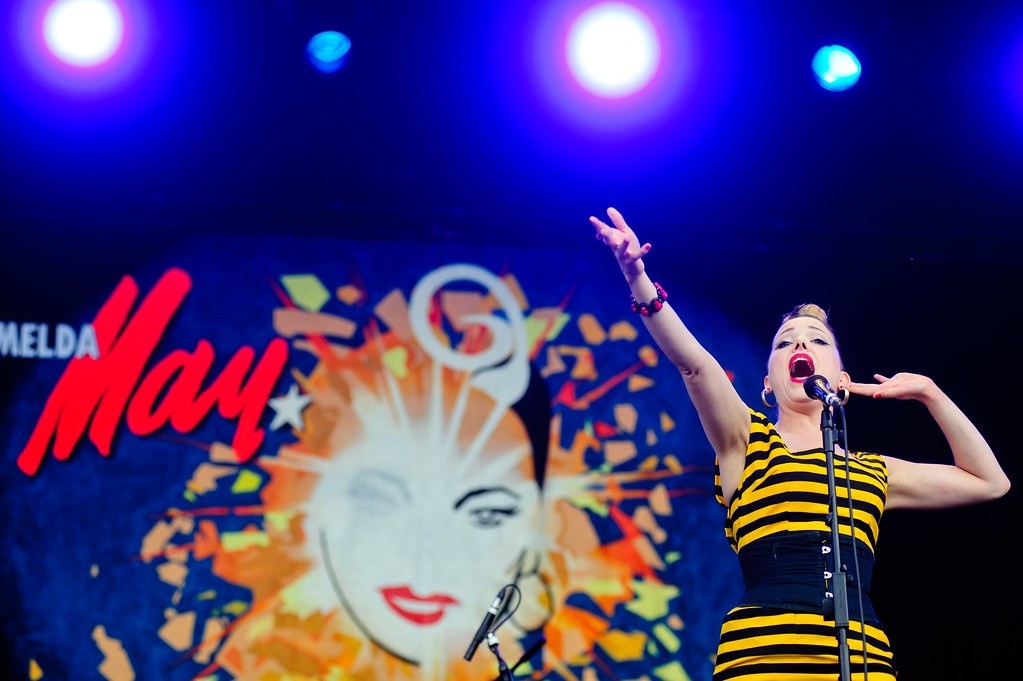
[803,375,843,410]
[463,587,506,662]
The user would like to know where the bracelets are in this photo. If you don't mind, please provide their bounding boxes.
[630,282,667,316]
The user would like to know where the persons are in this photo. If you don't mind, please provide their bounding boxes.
[590,208,1011,680]
